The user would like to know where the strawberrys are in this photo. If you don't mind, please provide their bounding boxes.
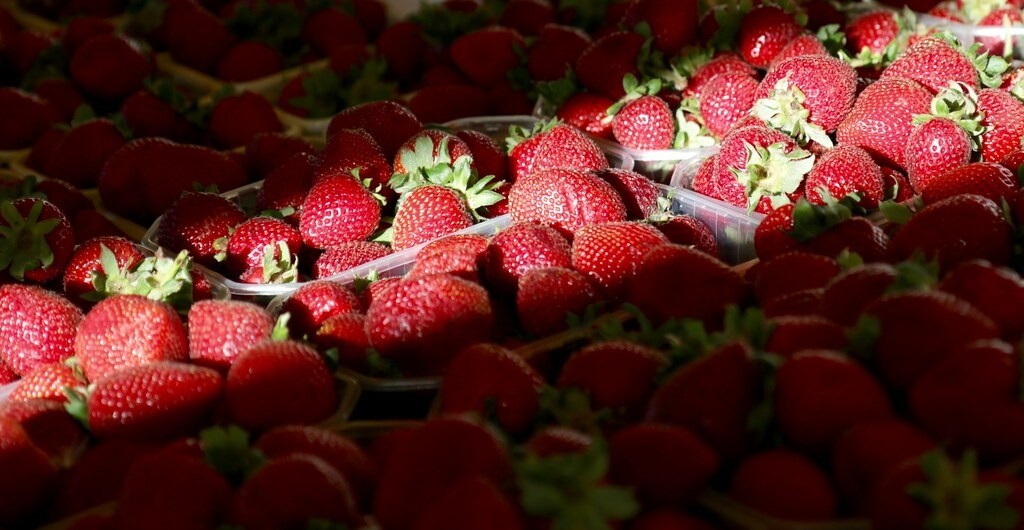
[0,0,1024,530]
